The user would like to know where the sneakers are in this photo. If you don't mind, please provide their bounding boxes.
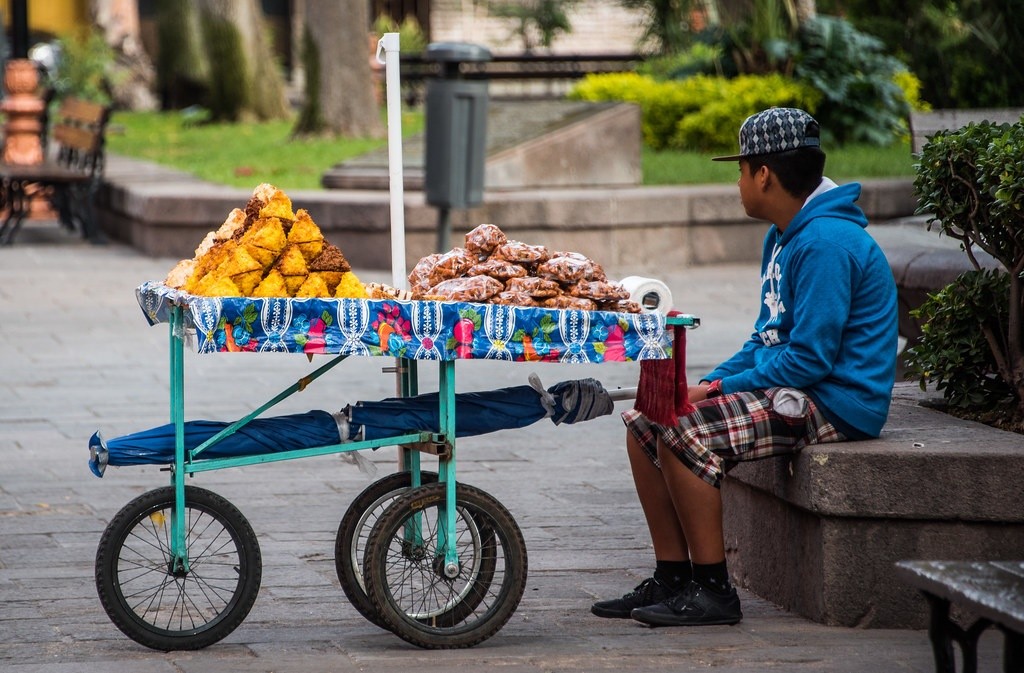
[590,574,676,617]
[631,578,744,625]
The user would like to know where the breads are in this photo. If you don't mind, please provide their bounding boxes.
[163,183,396,300]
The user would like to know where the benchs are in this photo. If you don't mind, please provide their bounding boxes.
[0,96,112,246]
[896,557,1024,672]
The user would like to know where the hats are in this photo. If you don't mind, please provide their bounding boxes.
[712,107,821,162]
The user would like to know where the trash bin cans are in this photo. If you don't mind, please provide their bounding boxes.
[425,42,488,208]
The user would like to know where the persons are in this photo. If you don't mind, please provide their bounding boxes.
[593,107,897,624]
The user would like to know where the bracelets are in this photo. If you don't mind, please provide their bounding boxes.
[706,379,721,399]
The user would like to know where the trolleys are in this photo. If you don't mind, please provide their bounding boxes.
[93,31,704,651]
